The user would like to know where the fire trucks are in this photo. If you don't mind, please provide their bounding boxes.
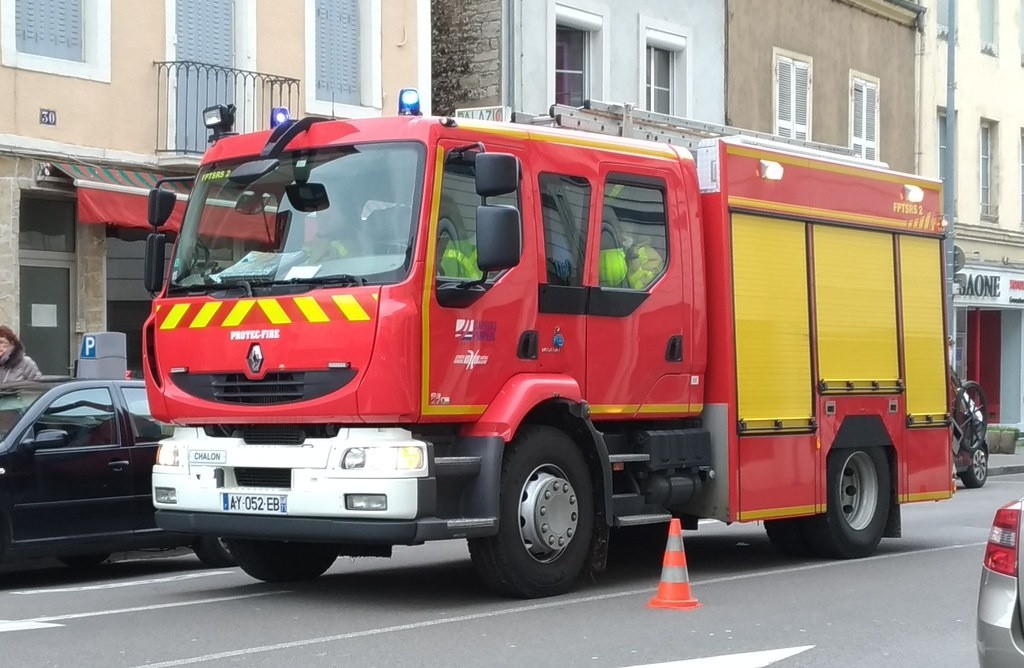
[140,86,953,602]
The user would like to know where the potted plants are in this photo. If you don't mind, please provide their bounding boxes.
[987,426,1020,453]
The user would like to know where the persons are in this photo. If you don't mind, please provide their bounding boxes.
[615,209,663,290]
[300,200,364,264]
[0,325,42,383]
[440,196,499,279]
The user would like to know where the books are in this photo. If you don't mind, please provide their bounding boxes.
[215,251,307,282]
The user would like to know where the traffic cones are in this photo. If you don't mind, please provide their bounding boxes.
[645,518,705,610]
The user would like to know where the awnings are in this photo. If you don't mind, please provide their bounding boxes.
[52,163,276,242]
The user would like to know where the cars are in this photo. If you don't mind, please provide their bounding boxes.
[0,379,237,572]
[976,495,1024,668]
